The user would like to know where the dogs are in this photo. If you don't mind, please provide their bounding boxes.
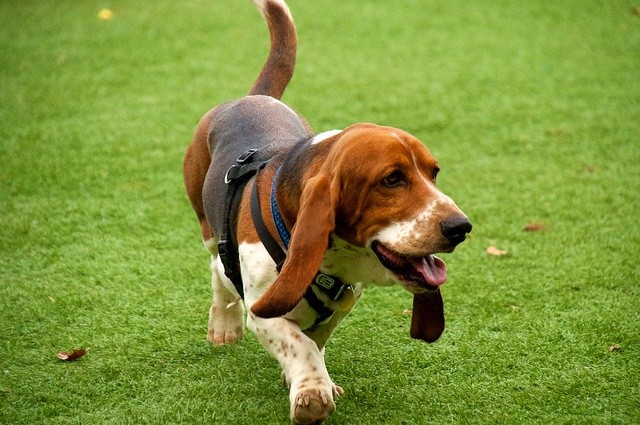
[181,0,472,425]
